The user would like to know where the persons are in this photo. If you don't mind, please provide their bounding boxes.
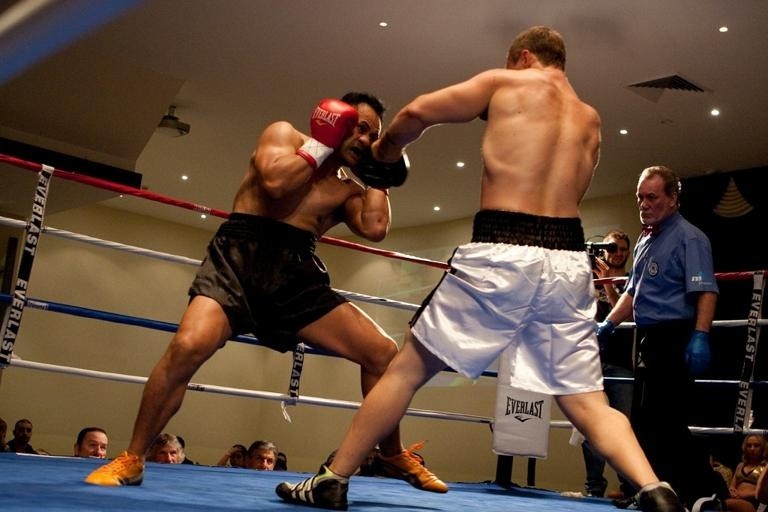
[272,30,690,512]
[707,435,768,512]
[83,92,451,493]
[581,230,634,499]
[595,166,721,512]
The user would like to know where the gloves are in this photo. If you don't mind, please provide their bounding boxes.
[591,317,614,354]
[683,326,712,383]
[292,97,360,173]
[349,143,410,191]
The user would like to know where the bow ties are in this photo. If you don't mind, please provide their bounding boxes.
[638,222,661,237]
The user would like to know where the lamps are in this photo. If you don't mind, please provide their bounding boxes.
[153,107,192,139]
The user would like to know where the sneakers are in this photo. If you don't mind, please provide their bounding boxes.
[368,438,450,495]
[628,479,689,512]
[80,449,148,490]
[271,463,351,511]
